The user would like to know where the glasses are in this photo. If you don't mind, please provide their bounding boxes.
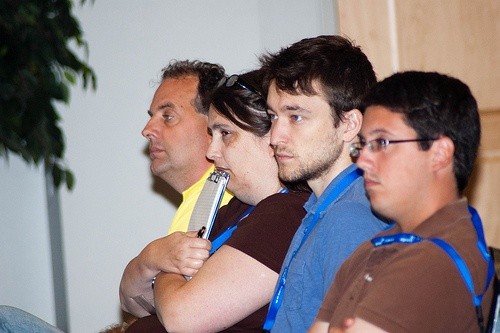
[349,136,438,156]
[215,74,266,98]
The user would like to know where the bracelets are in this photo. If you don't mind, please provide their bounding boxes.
[151,276,157,291]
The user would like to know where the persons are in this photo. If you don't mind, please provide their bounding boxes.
[308,72,496,333]
[1,59,236,333]
[257,35,377,332]
[119,71,309,333]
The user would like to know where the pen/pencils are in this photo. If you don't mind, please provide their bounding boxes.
[196,226,206,238]
